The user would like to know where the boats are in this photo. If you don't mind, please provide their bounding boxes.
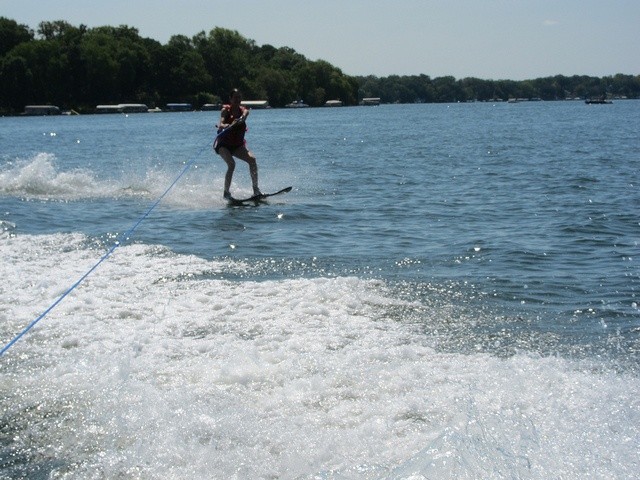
[585,96,613,104]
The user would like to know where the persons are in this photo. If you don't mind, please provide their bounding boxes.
[213,89,262,199]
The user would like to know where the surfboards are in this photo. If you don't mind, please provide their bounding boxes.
[228,186,292,204]
